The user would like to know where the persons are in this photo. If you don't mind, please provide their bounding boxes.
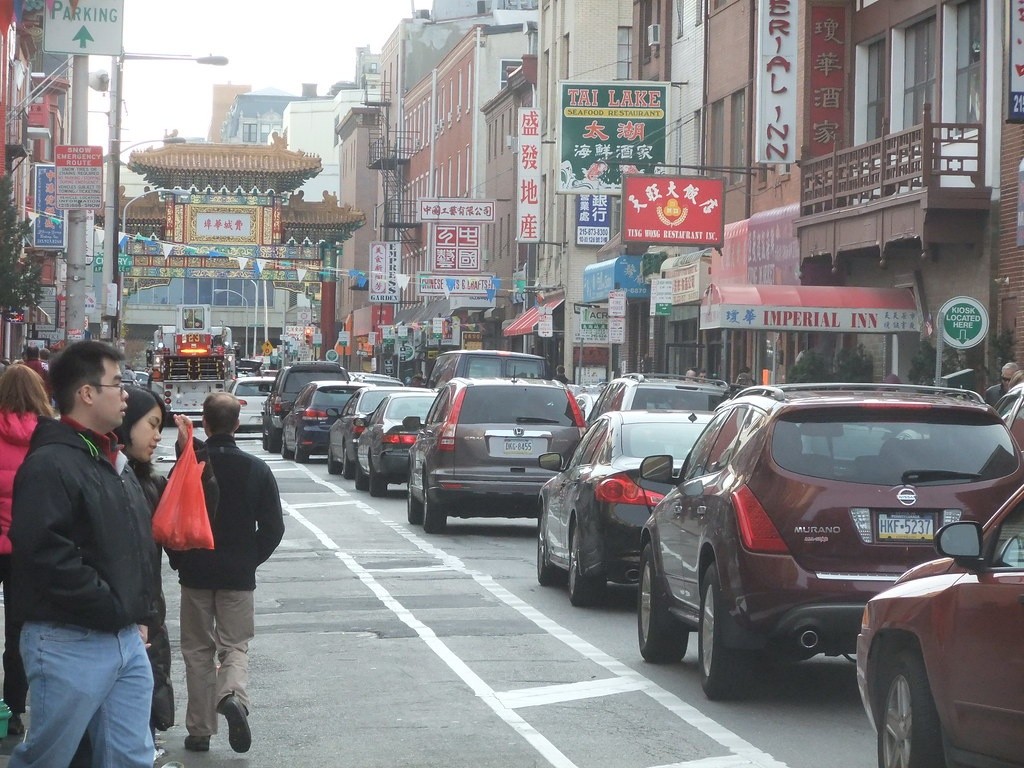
[406,370,426,387]
[552,365,569,387]
[255,362,264,392]
[685,366,698,382]
[733,365,757,387]
[877,373,901,392]
[695,368,707,384]
[0,339,220,768]
[984,360,1024,410]
[162,393,285,753]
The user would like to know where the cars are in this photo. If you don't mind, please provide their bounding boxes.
[227,376,276,433]
[353,392,439,497]
[853,482,1024,768]
[277,380,377,464]
[325,385,431,480]
[569,382,610,421]
[535,407,717,608]
[121,367,150,390]
[237,356,278,377]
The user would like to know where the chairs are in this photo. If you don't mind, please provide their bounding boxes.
[880,437,945,480]
[775,424,837,481]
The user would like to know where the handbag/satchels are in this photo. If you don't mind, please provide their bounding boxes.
[150,424,216,551]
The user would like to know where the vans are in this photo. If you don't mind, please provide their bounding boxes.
[424,348,548,389]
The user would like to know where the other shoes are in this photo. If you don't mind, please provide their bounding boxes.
[8,712,25,735]
[185,734,211,752]
[223,695,252,753]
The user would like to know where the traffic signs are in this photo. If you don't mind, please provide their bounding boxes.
[934,294,990,350]
[42,0,125,57]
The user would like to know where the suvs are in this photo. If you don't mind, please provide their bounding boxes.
[399,376,590,533]
[636,380,1024,702]
[258,361,351,453]
[583,369,738,429]
[994,380,1024,456]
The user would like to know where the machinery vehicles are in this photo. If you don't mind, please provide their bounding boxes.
[144,304,241,425]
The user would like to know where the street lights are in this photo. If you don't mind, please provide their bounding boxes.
[100,136,187,342]
[213,288,248,359]
[112,51,232,345]
[117,188,191,353]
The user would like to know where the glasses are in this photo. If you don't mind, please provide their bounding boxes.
[78,382,125,394]
[1001,373,1011,381]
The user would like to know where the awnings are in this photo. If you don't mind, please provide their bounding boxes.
[502,296,565,338]
[10,291,52,325]
[581,254,651,305]
[699,283,922,386]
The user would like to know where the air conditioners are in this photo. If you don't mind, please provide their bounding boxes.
[647,24,660,47]
